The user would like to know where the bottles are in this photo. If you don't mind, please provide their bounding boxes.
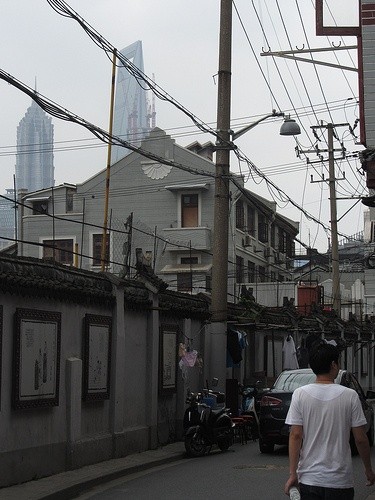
[289,486,301,500]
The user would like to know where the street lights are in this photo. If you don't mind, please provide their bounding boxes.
[228,109,301,142]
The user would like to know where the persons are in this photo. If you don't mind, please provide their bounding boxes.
[283,343,375,500]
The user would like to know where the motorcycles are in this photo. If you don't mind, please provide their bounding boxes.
[182,378,235,457]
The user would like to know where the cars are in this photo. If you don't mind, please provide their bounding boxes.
[258,367,375,457]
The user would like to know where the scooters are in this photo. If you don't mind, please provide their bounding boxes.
[234,380,272,439]
